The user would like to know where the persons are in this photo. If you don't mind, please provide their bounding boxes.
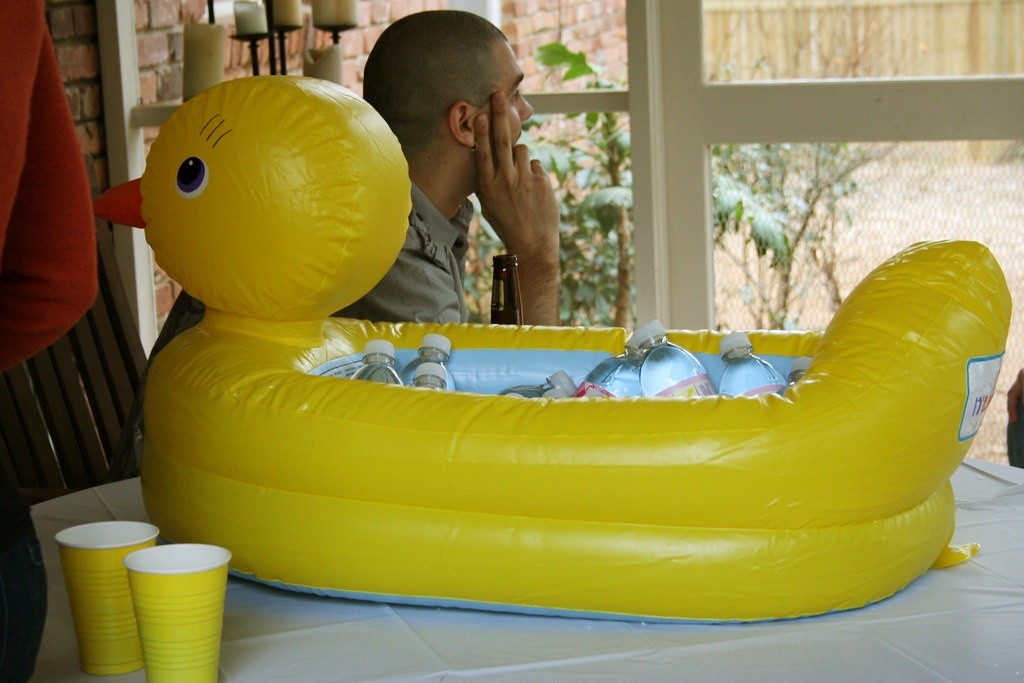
[1007,372,1023,424]
[111,9,560,479]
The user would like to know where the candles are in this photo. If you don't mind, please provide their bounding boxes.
[181,22,226,105]
[232,0,269,35]
[311,0,360,28]
[301,43,343,88]
[271,0,303,27]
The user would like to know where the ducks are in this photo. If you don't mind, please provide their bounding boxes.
[94,75,1013,625]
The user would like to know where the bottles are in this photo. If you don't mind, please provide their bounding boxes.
[633,318,717,400]
[717,331,786,401]
[398,333,456,389]
[575,330,656,399]
[349,339,404,386]
[490,254,524,329]
[775,356,812,400]
[499,369,581,398]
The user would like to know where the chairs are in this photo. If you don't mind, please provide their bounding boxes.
[0,198,154,547]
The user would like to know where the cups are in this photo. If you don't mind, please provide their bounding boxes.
[122,540,233,682]
[54,520,161,673]
